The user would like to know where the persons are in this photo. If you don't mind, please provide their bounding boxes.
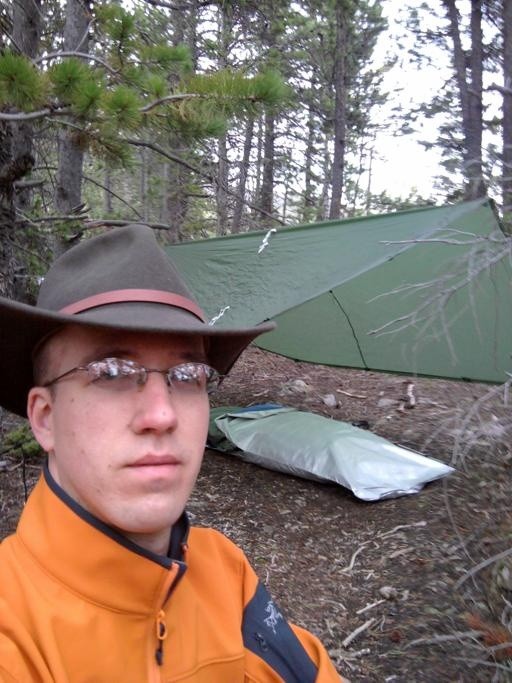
[1,220,353,683]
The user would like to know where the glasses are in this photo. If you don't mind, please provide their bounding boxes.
[43,357,220,394]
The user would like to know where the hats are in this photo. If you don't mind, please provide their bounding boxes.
[0,224,277,419]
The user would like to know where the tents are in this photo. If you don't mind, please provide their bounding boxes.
[163,194,509,384]
